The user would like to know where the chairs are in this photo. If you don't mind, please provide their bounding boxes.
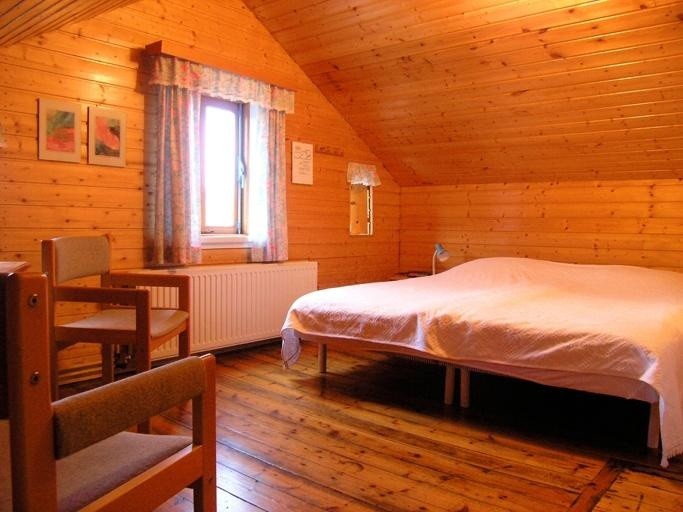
[41,236,191,434]
[1,271,216,510]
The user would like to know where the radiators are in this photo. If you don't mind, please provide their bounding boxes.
[126,261,321,366]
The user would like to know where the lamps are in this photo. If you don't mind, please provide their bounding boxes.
[432,245,448,273]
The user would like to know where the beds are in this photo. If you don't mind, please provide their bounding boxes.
[283,257,683,449]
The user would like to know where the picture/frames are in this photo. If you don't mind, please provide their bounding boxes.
[37,97,126,167]
